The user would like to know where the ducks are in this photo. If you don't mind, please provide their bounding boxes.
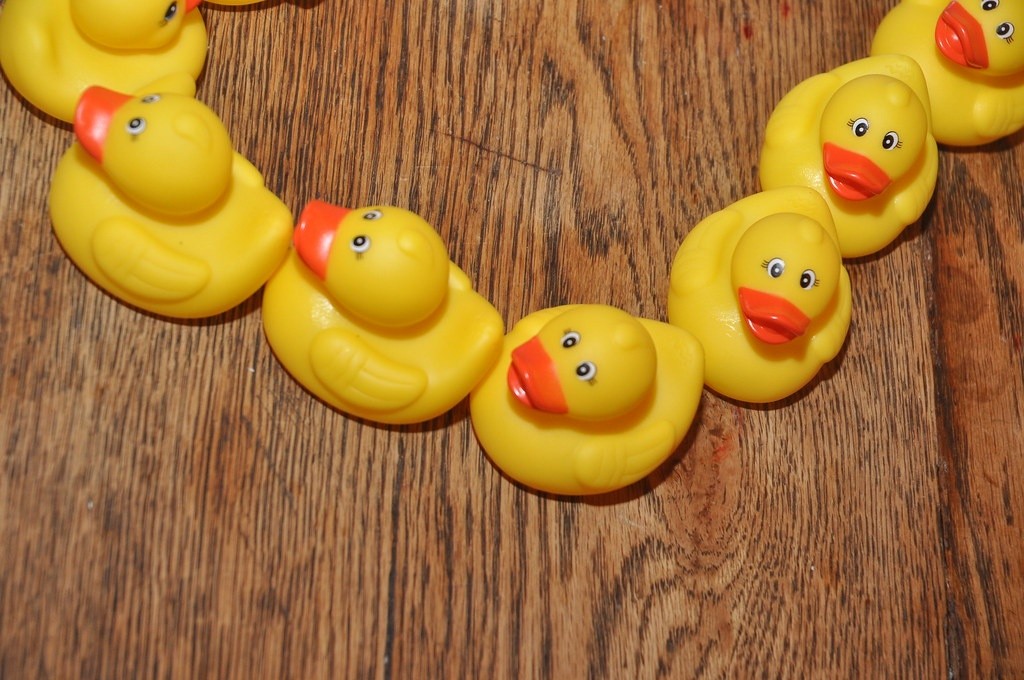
[2,0,1021,501]
